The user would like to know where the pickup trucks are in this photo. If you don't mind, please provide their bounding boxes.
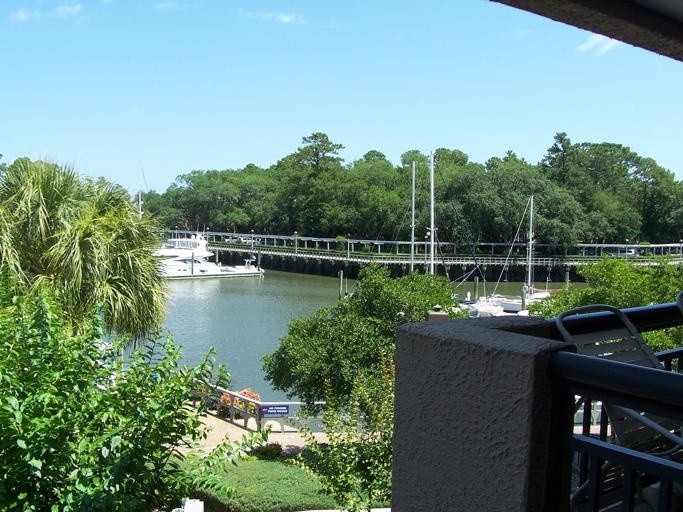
[224,237,256,245]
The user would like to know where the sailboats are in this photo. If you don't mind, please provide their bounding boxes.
[346,150,556,323]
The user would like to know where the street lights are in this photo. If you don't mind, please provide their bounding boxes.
[174,226,179,237]
[250,229,255,249]
[294,232,298,253]
[206,227,211,242]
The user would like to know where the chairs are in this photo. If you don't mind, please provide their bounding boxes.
[556,302,683,512]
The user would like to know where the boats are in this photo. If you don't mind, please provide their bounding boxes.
[151,238,210,264]
[155,251,265,280]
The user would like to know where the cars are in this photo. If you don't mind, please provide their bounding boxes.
[607,249,638,257]
